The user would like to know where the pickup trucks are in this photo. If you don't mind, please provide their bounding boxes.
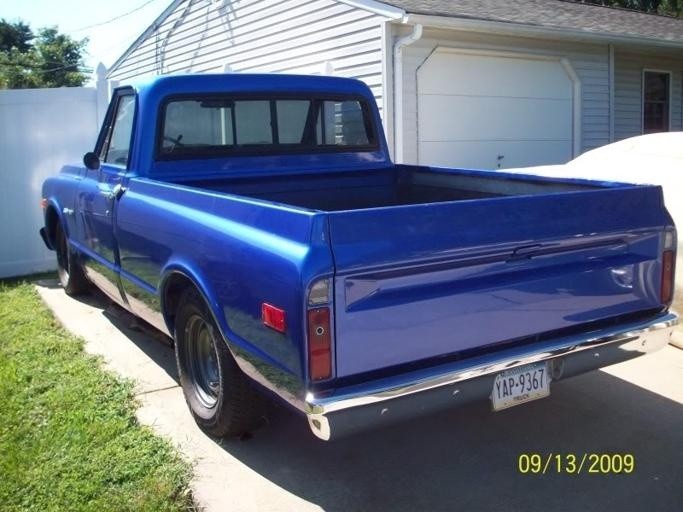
[38,69,682,443]
